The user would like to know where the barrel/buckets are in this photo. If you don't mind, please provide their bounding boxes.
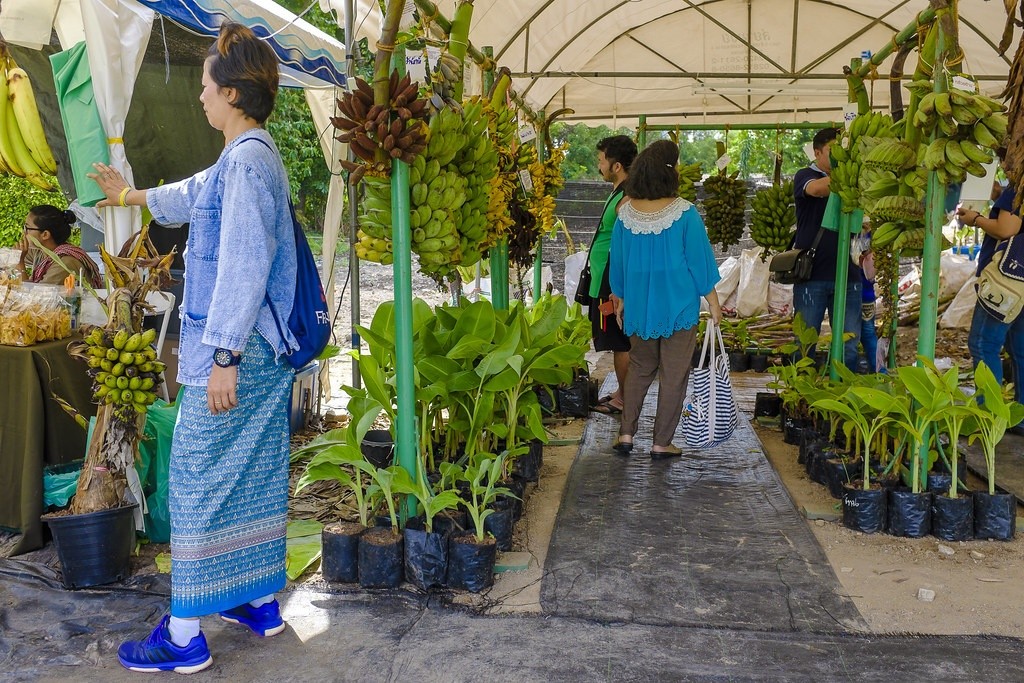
[359,429,394,469]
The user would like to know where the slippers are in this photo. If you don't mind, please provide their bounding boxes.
[592,394,627,414]
[651,445,680,459]
[613,435,632,452]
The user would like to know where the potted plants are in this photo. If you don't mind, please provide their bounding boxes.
[692,317,775,372]
[755,312,1024,542]
[293,290,597,591]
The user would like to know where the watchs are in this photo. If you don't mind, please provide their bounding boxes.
[213,348,241,368]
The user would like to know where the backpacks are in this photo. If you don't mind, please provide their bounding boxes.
[232,137,330,365]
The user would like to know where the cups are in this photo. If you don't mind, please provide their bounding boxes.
[59,287,83,332]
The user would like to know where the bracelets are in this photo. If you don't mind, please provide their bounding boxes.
[973,215,983,226]
[118,187,132,207]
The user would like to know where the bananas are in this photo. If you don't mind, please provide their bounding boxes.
[329,51,570,277]
[85,325,167,413]
[676,161,798,252]
[827,80,1011,258]
[0,40,59,192]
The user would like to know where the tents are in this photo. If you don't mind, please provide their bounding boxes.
[0,1,361,389]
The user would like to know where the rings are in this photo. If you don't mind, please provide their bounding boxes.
[214,402,221,404]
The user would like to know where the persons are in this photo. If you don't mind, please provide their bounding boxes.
[795,128,887,375]
[589,134,638,417]
[12,204,102,289]
[957,147,1024,437]
[87,23,301,675]
[612,140,723,459]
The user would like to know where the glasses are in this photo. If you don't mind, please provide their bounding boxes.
[24,225,46,235]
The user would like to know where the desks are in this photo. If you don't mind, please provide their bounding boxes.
[0,333,98,558]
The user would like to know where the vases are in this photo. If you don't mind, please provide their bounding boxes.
[40,503,139,589]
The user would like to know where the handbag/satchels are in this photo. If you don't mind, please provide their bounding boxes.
[573,265,594,306]
[973,253,1024,323]
[681,319,738,447]
[769,251,814,285]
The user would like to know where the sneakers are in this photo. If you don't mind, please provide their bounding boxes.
[221,596,285,636]
[117,614,213,675]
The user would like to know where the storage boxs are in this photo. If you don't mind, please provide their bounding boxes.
[290,364,319,433]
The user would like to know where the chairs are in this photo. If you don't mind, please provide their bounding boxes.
[141,291,176,403]
